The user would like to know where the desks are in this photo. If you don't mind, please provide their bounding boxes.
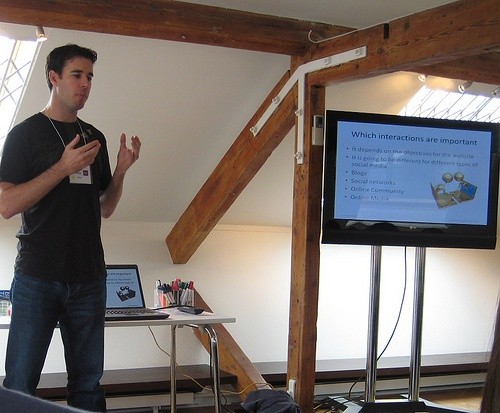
[0,306,236,413]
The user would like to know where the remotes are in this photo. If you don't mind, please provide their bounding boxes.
[178,306,204,315]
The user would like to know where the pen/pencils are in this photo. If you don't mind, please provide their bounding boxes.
[155,278,193,307]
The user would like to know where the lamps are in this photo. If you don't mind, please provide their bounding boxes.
[35,26,47,43]
[457,81,473,93]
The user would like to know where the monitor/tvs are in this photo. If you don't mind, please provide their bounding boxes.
[320,110,500,250]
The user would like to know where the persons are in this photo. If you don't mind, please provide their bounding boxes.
[0,44,141,413]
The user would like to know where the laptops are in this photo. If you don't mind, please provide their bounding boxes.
[104,265,170,321]
